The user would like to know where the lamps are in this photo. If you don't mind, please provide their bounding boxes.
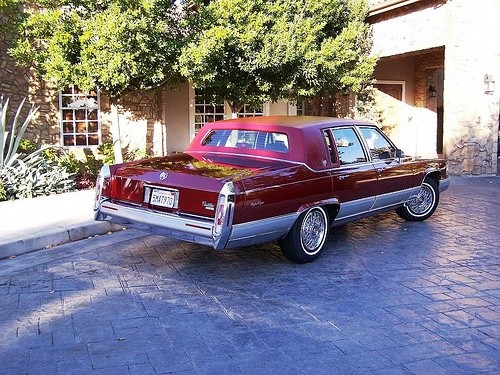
[484,73,495,95]
[428,85,437,97]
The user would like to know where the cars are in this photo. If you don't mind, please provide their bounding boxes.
[93,113,451,264]
